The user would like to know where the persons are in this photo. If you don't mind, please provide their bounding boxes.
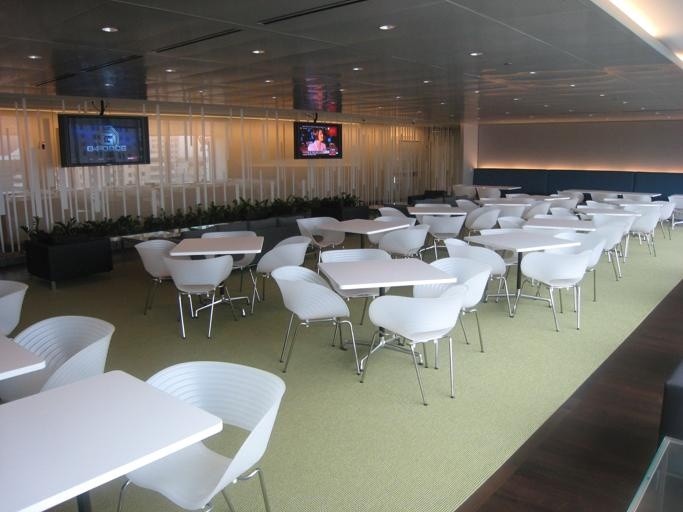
[306,127,327,152]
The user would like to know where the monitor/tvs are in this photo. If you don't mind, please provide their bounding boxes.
[52,48,147,100]
[57,114,150,168]
[292,122,343,159]
[292,67,342,113]
